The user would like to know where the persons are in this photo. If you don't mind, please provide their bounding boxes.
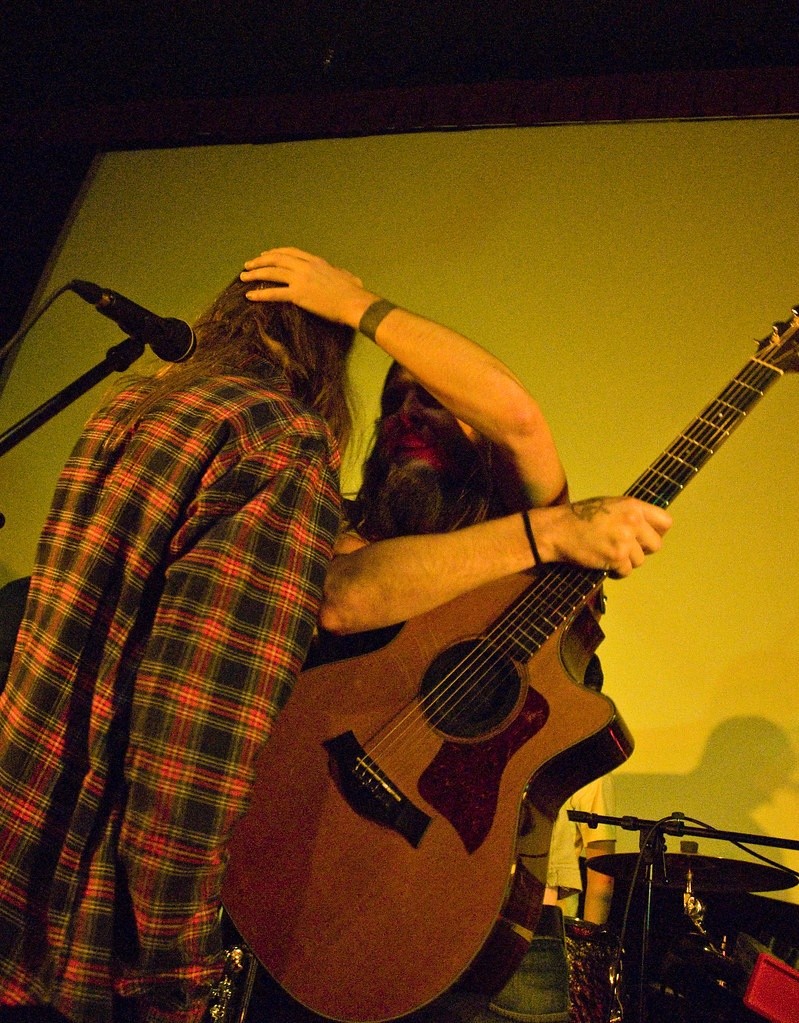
[544,771,616,924]
[0,268,359,1023]
[240,245,673,1023]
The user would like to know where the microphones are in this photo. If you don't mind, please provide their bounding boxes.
[67,280,197,363]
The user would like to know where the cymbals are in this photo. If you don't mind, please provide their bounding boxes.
[585,850,799,892]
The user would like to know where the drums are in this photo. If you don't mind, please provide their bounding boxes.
[567,916,624,1023]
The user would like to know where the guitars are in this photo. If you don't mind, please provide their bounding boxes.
[214,304,799,1023]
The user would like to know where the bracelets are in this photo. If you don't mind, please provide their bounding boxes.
[522,510,542,566]
[359,299,398,344]
